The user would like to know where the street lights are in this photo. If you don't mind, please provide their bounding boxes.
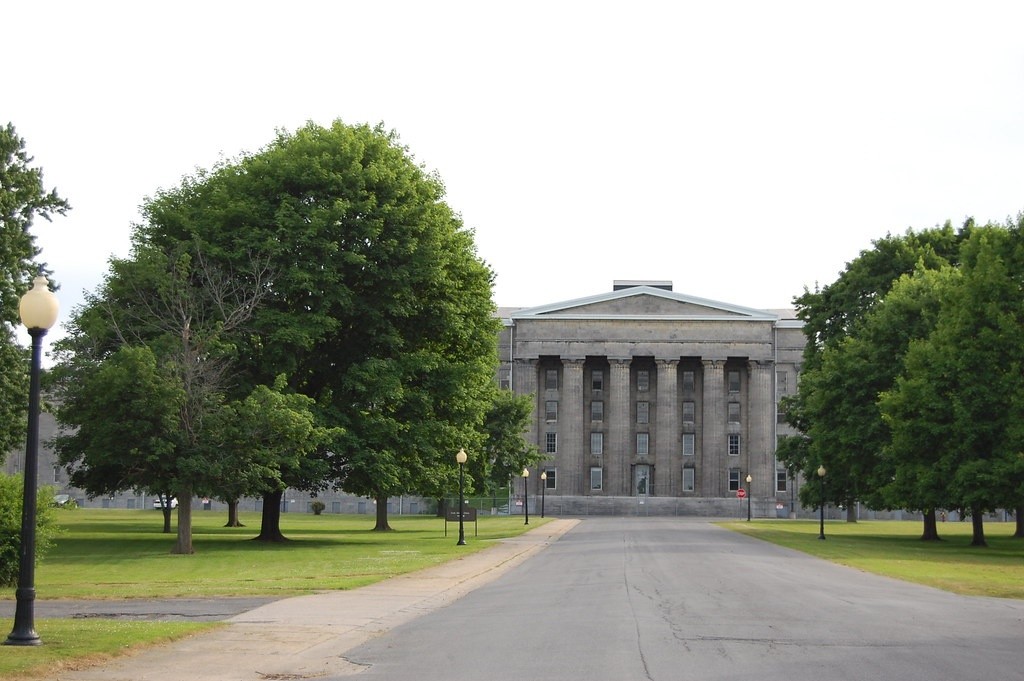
[521,468,530,525]
[746,474,752,521]
[817,464,826,539]
[455,448,468,545]
[541,472,547,518]
[3,275,59,648]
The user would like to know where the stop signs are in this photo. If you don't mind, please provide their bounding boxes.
[737,489,746,498]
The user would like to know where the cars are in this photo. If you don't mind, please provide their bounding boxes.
[154,494,179,511]
[49,494,80,508]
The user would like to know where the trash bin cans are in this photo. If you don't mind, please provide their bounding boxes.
[204,500,211,510]
[776,505,790,519]
